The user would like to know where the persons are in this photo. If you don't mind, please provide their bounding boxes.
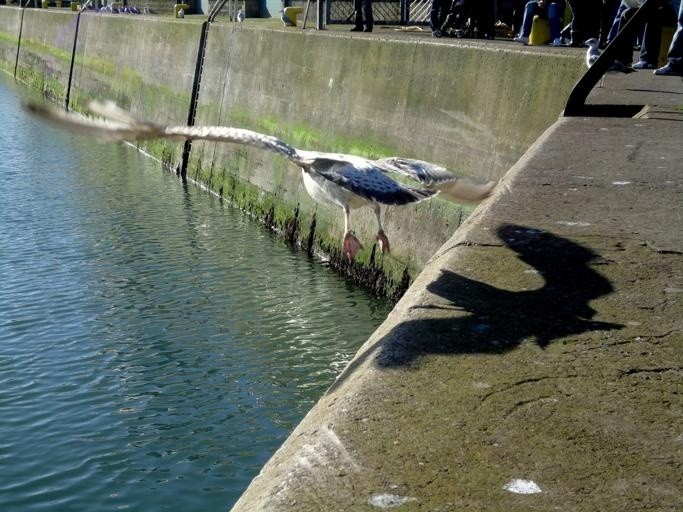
[429,0,682,77]
[349,0,375,32]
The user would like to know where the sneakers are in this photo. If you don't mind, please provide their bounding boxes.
[633,61,658,69]
[514,36,528,43]
[654,66,681,76]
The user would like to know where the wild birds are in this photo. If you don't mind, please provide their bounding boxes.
[236,9,245,23]
[583,36,638,89]
[277,9,298,29]
[177,8,185,18]
[18,94,497,266]
[82,3,158,15]
